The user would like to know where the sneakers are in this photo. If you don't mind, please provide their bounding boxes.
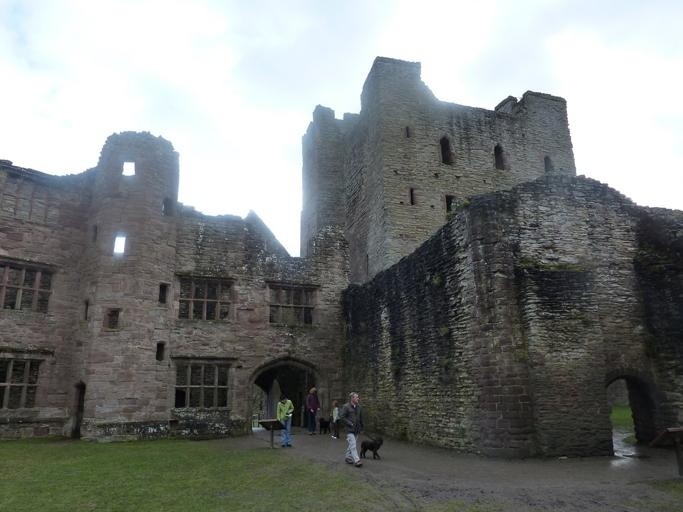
[281,442,291,447]
[355,460,362,466]
[345,458,354,464]
[332,436,339,439]
[308,431,316,435]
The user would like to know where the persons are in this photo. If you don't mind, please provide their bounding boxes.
[276,394,295,448]
[339,391,367,467]
[329,400,341,440]
[305,387,321,435]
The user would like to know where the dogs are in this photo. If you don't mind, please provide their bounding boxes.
[318,417,332,435]
[360,436,383,459]
